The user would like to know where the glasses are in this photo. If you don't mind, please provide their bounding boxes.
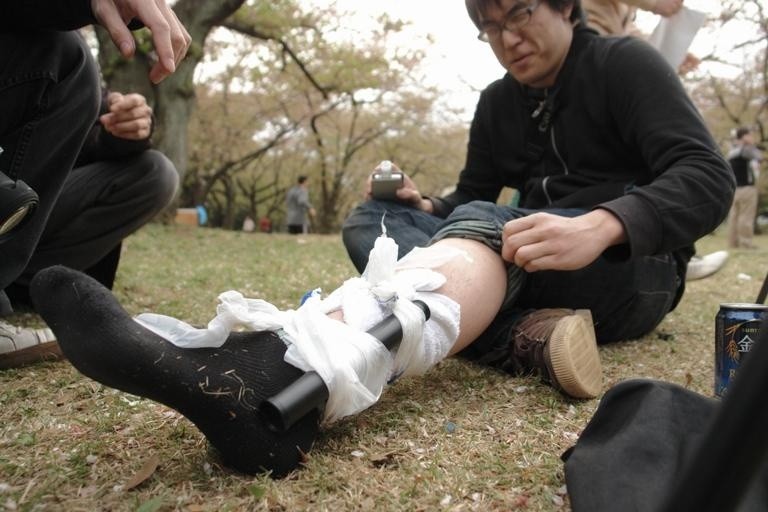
[477,1,543,43]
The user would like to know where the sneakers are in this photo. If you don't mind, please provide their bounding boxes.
[496,304,603,402]
[0,318,66,373]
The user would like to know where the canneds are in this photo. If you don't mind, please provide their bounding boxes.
[715,302,768,401]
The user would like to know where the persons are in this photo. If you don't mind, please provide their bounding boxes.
[1,0,191,367]
[32,0,737,477]
[284,173,317,236]
[575,0,730,284]
[723,128,767,252]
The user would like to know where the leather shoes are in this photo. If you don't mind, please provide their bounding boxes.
[685,249,730,284]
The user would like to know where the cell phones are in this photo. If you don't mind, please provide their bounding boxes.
[372,172,404,201]
